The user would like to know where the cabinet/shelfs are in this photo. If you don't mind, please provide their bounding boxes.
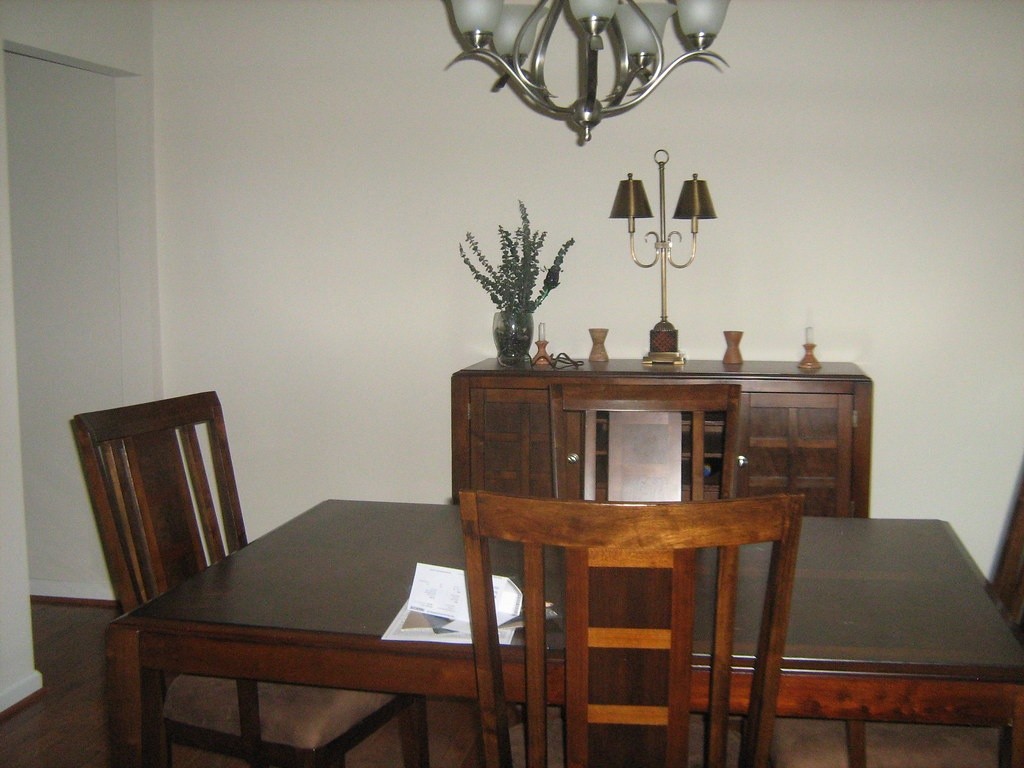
[451,357,874,519]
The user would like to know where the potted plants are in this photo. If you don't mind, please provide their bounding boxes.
[458,198,576,364]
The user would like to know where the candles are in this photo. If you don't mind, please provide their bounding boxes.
[539,321,546,342]
[805,326,814,344]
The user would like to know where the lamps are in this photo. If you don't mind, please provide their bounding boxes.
[447,0,731,141]
[608,149,719,365]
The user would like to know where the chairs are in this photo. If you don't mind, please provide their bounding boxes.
[458,488,805,768]
[72,391,432,768]
[766,474,1024,768]
[548,381,742,502]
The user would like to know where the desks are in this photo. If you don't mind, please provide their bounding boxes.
[102,497,1024,768]
